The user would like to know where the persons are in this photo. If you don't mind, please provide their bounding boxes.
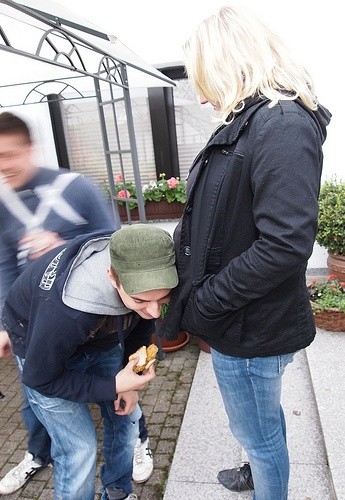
[159,7,331,500]
[4,226,179,500]
[0,111,153,493]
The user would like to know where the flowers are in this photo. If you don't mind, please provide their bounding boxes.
[309,273,345,316]
[102,173,186,210]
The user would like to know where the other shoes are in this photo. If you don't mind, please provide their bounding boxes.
[99,486,137,500]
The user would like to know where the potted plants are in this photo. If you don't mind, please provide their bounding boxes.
[150,305,190,355]
[315,182,345,289]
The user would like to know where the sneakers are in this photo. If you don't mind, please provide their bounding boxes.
[0,450,48,495]
[217,462,254,492]
[132,436,154,483]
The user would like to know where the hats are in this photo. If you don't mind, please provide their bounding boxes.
[109,224,178,295]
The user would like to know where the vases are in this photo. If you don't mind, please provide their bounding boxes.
[310,300,345,332]
[118,198,186,221]
